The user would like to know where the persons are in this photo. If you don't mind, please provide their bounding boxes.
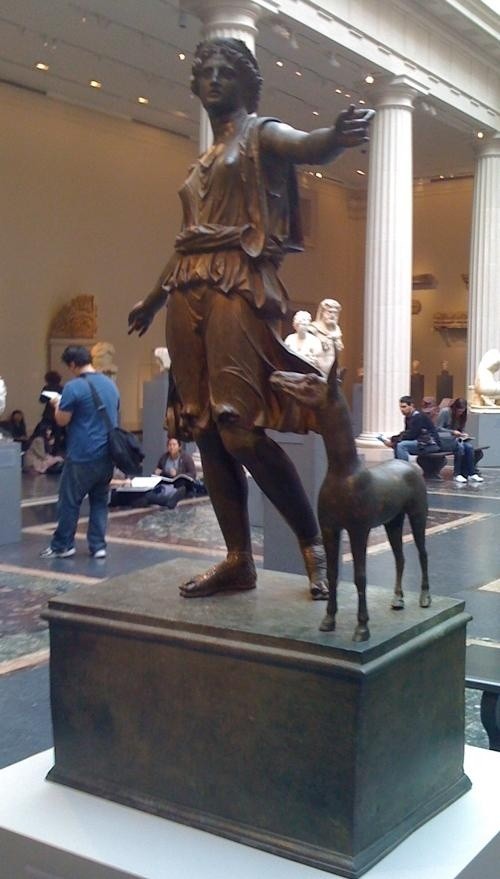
[36,344,122,563]
[433,396,486,483]
[107,483,188,509]
[125,34,381,603]
[38,370,63,452]
[280,307,319,371]
[152,439,197,493]
[22,420,65,475]
[4,409,31,468]
[379,396,441,464]
[307,294,347,351]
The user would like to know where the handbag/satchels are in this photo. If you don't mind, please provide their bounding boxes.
[107,427,145,477]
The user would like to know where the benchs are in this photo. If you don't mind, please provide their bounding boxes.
[422,446,490,475]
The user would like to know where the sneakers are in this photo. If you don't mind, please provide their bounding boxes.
[89,546,106,559]
[39,543,76,559]
[452,474,468,483]
[467,474,484,482]
